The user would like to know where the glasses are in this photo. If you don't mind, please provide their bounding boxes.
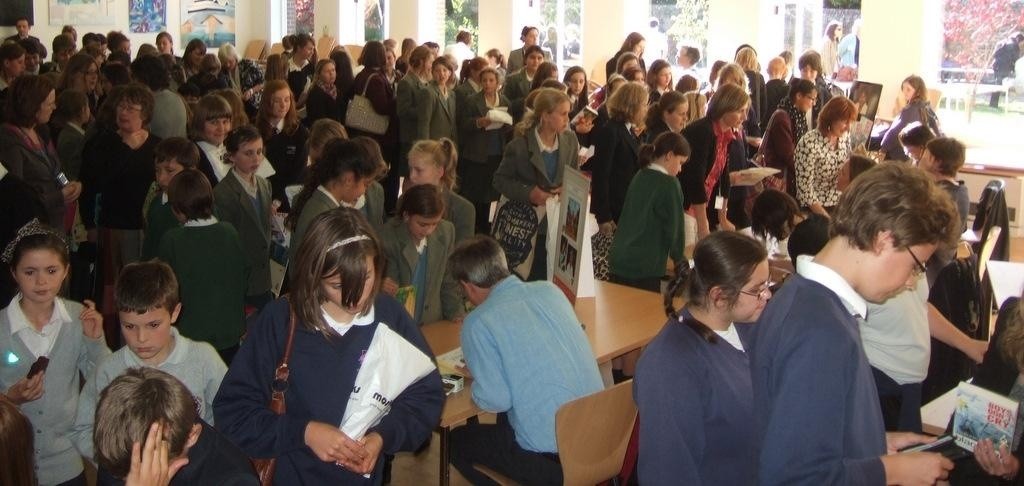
[906,246,927,275]
[117,102,140,113]
[740,273,772,301]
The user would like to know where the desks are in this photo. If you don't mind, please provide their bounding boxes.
[382,272,688,486]
[982,259,1023,317]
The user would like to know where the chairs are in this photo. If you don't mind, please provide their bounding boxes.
[956,176,1009,252]
[467,376,638,486]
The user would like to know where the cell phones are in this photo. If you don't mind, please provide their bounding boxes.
[56,173,70,187]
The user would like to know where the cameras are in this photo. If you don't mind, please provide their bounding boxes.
[27,356,50,379]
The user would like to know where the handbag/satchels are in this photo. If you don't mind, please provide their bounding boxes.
[491,191,552,280]
[345,94,391,134]
[591,228,618,281]
[745,158,785,223]
[247,396,287,486]
